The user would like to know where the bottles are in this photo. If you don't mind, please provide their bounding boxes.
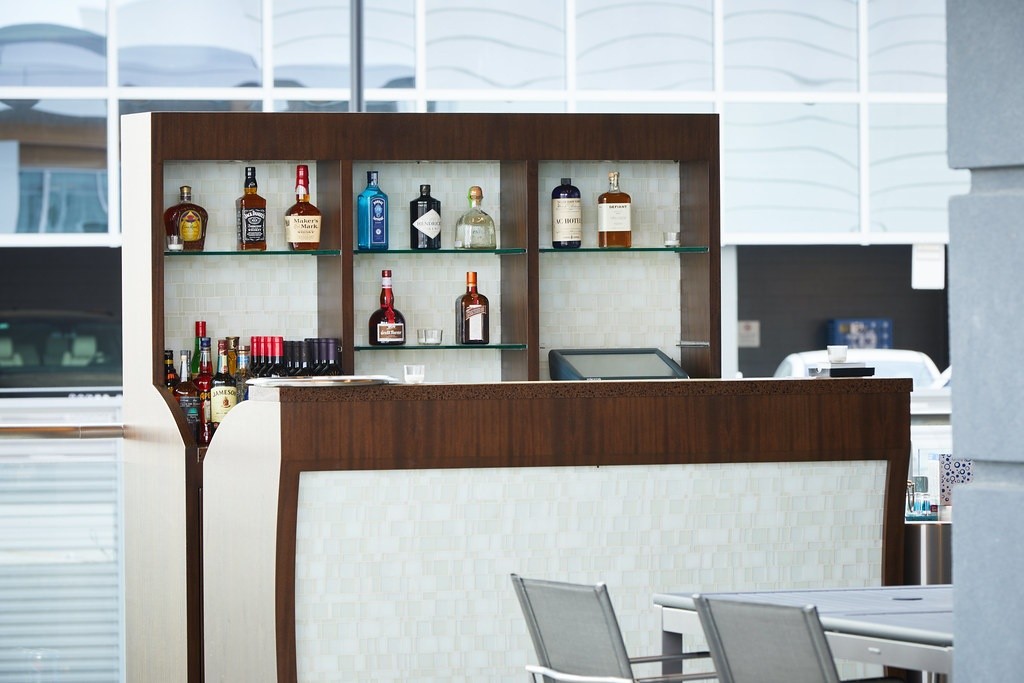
[551,177,582,250]
[598,172,632,248]
[235,166,267,252]
[165,320,343,446]
[904,492,932,516]
[164,186,208,253]
[283,165,323,250]
[454,186,498,251]
[454,272,490,345]
[409,184,442,251]
[357,170,389,251]
[369,270,406,346]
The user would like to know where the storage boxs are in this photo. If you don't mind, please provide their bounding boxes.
[826,318,894,348]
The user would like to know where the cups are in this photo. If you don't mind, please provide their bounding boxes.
[165,235,185,253]
[416,328,425,345]
[827,345,848,364]
[424,328,443,346]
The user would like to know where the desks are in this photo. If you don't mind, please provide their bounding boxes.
[653,583,956,683]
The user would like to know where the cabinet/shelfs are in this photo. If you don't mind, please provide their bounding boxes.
[120,109,723,683]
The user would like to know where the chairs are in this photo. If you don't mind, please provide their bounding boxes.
[691,592,841,683]
[508,571,717,683]
